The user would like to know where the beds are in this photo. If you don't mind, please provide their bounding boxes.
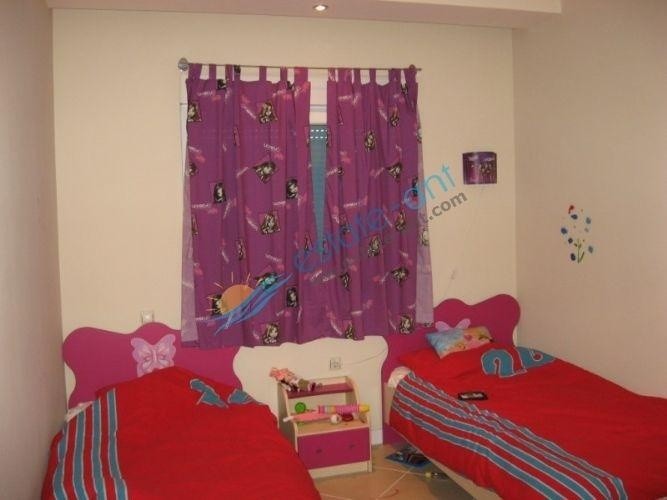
[40,323,324,500]
[382,294,667,500]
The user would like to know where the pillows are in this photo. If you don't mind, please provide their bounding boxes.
[424,325,494,360]
[400,346,440,371]
[96,383,119,397]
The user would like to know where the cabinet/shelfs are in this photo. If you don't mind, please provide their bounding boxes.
[277,375,373,479]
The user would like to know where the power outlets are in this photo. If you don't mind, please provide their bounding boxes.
[330,358,341,370]
